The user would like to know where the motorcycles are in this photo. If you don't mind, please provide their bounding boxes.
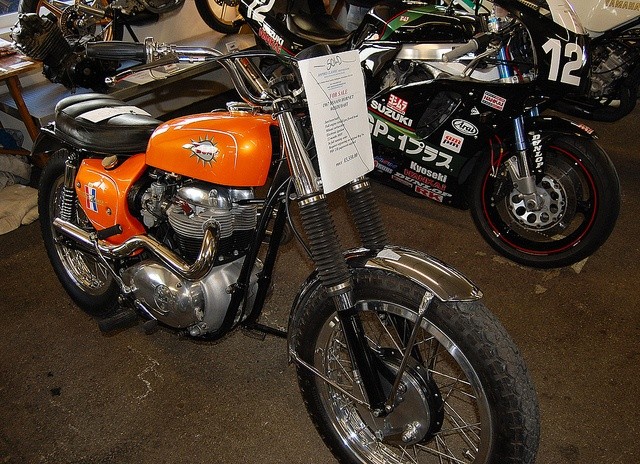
[225,0,620,270]
[18,0,248,43]
[30,5,541,464]
[547,0,640,124]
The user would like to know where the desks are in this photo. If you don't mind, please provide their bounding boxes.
[1,52,40,144]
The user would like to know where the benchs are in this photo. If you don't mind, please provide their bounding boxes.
[1,30,255,130]
